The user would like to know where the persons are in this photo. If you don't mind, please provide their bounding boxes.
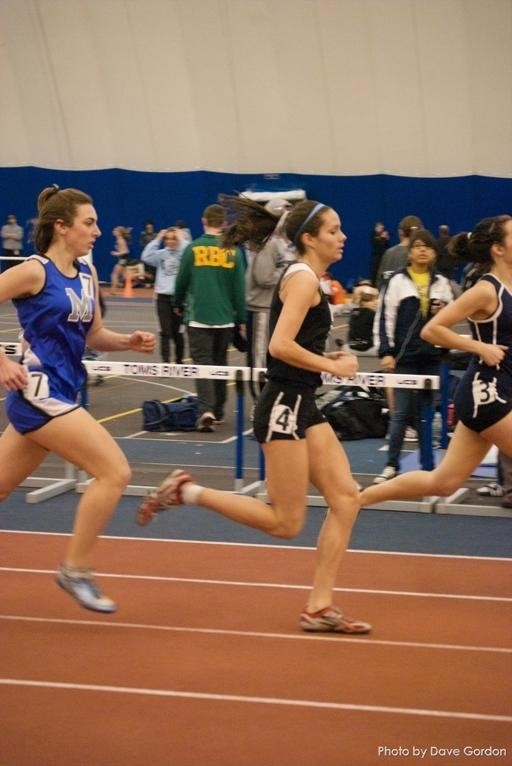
[0,184,160,616]
[1,194,484,447]
[131,194,374,638]
[354,213,510,515]
[371,232,456,487]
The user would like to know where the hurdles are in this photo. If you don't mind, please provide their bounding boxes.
[76,361,266,501]
[435,358,511,517]
[0,258,27,359]
[15,458,78,503]
[252,372,443,515]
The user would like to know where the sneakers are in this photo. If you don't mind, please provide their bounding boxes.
[373,467,396,483]
[136,469,192,525]
[300,604,372,634]
[56,562,116,612]
[214,418,224,425]
[197,412,216,433]
[477,481,511,497]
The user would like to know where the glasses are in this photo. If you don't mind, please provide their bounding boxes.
[413,243,434,248]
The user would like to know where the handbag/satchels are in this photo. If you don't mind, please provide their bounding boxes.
[141,396,201,432]
[319,386,391,439]
